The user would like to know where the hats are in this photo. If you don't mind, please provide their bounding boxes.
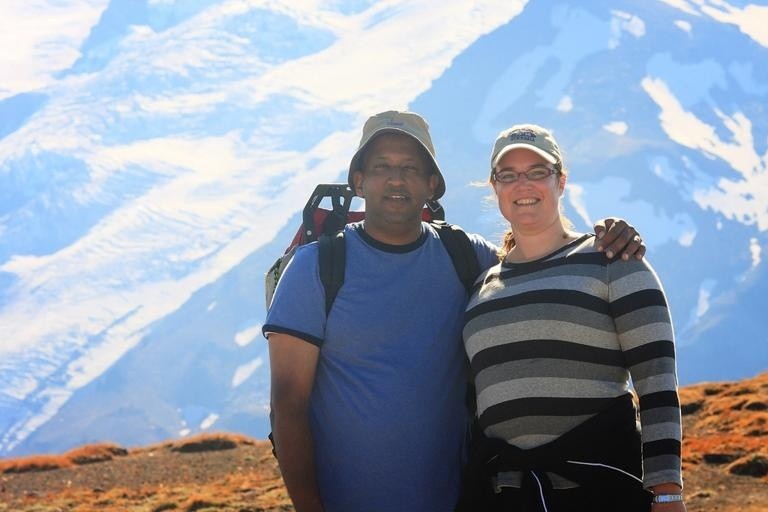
[348,110,446,203]
[490,124,562,168]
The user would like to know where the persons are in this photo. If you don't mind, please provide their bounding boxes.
[459,120,687,511]
[261,109,646,511]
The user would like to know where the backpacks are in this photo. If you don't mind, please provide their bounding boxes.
[264,184,483,322]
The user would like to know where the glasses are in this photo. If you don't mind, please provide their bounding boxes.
[494,165,558,183]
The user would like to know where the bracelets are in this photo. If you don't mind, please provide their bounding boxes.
[653,493,684,502]
